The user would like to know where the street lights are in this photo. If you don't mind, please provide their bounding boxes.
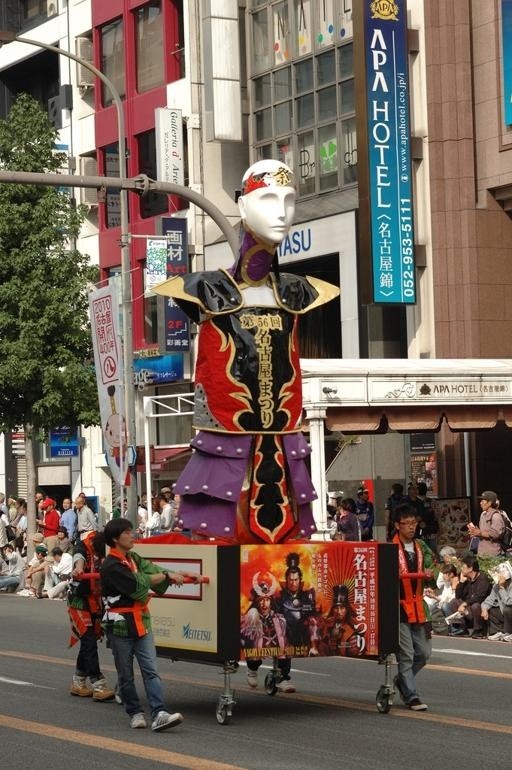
[0,30,138,534]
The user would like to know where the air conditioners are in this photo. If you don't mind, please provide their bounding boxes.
[46,0,58,19]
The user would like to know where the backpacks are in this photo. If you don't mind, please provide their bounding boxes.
[489,510,512,552]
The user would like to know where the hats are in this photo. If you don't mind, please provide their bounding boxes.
[36,544,48,555]
[41,498,55,509]
[356,487,368,495]
[161,487,171,493]
[33,533,44,542]
[476,491,497,501]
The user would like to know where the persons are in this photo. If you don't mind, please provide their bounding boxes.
[95,518,204,733]
[381,506,439,711]
[65,530,115,703]
[144,161,342,542]
[325,481,512,641]
[1,481,181,601]
[245,660,296,693]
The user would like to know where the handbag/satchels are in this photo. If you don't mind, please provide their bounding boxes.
[5,524,16,541]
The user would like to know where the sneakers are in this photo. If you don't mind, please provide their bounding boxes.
[70,684,91,697]
[245,668,259,688]
[471,630,488,638]
[93,688,114,701]
[129,713,146,729]
[17,589,44,598]
[150,711,183,731]
[449,629,469,636]
[276,680,297,693]
[405,698,428,711]
[489,632,512,642]
